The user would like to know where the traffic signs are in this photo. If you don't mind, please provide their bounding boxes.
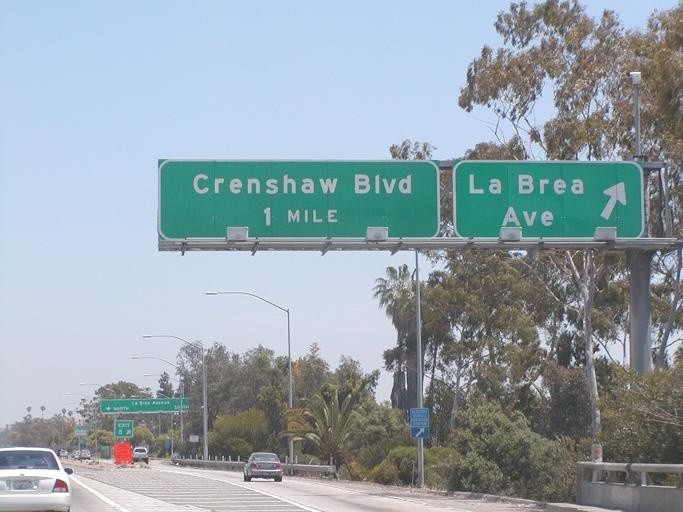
[450,157,651,244]
[97,398,190,416]
[153,158,444,243]
[112,418,136,440]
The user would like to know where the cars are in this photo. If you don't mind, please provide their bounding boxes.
[242,450,282,483]
[0,445,72,512]
[58,449,91,460]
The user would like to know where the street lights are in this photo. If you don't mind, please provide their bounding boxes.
[63,382,115,454]
[142,333,209,461]
[127,354,192,457]
[206,290,295,473]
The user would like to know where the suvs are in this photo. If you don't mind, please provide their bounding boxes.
[132,446,148,464]
